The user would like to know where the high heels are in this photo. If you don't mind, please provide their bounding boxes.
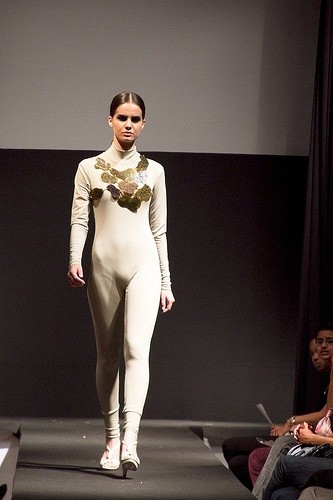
[120,442,139,478]
[99,441,120,470]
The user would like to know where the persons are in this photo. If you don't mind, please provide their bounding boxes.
[222,320,333,500]
[67,91,177,479]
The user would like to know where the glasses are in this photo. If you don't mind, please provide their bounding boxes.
[315,338,333,346]
